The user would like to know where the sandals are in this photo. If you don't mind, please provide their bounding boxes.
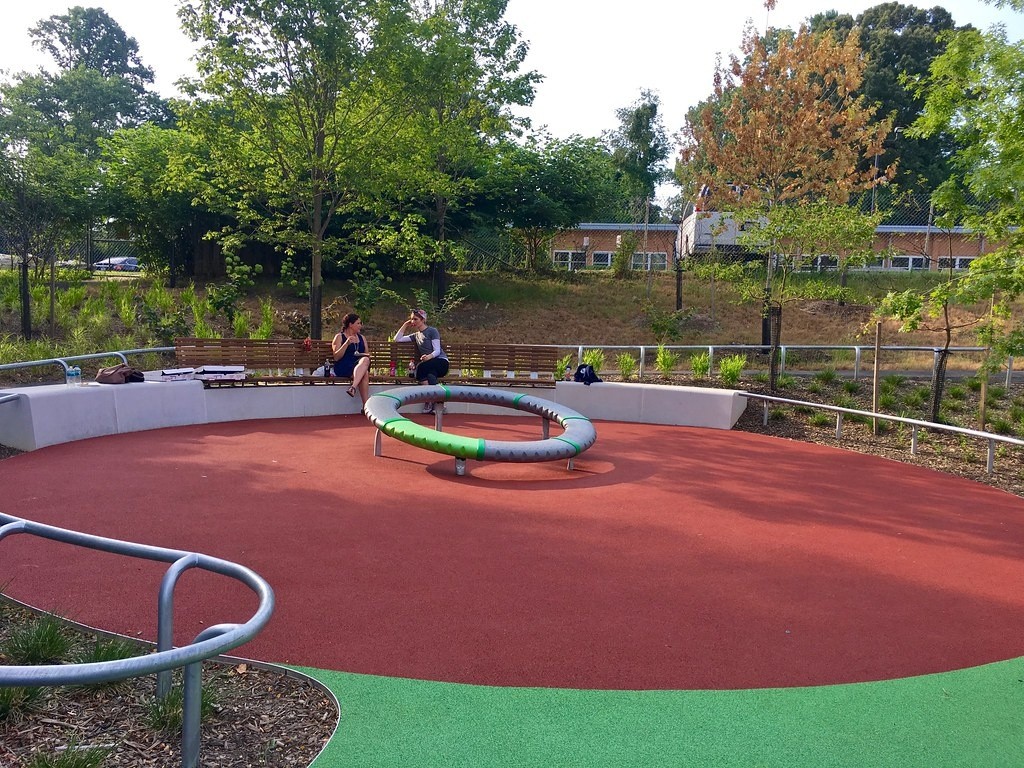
[361,409,365,414]
[346,386,357,397]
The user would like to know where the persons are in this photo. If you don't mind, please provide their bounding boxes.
[393,308,450,415]
[332,313,370,414]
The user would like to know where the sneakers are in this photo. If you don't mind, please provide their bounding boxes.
[422,401,432,413]
[431,405,447,415]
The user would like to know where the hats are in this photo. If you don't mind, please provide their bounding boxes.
[410,309,427,323]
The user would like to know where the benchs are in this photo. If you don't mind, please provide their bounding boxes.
[171,336,560,389]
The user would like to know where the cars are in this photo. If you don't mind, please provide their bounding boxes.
[95,256,145,272]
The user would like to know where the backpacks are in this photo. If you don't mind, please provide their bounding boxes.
[574,364,603,385]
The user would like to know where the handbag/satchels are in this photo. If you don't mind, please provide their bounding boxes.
[95,364,144,384]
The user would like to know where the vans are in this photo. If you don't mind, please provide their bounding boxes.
[671,180,776,266]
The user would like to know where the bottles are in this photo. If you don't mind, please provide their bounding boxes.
[74,365,81,387]
[566,366,571,381]
[409,360,415,377]
[324,359,330,376]
[66,366,75,387]
[389,359,396,377]
[398,360,403,376]
